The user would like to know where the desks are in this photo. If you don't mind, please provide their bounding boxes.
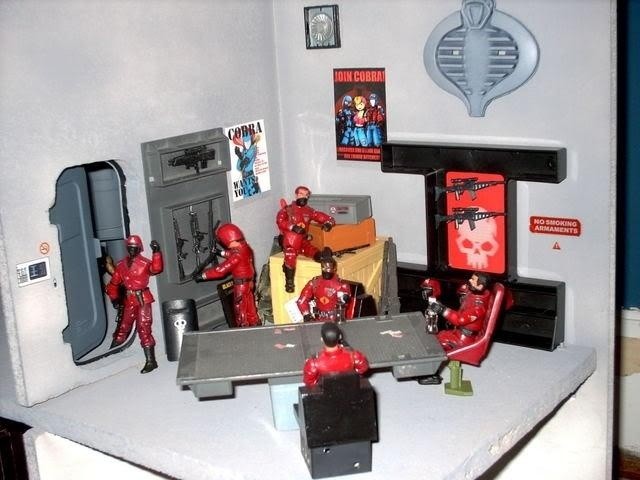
[18,336,598,480]
